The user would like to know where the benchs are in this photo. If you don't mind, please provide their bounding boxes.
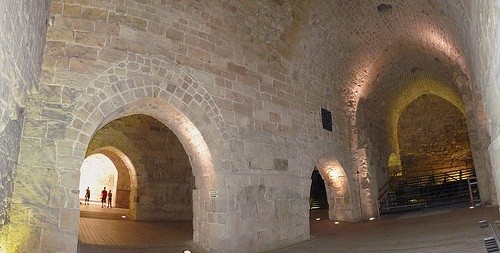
[373,161,476,217]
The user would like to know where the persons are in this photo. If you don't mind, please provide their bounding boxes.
[101,186,108,208]
[107,190,113,208]
[84,186,91,205]
